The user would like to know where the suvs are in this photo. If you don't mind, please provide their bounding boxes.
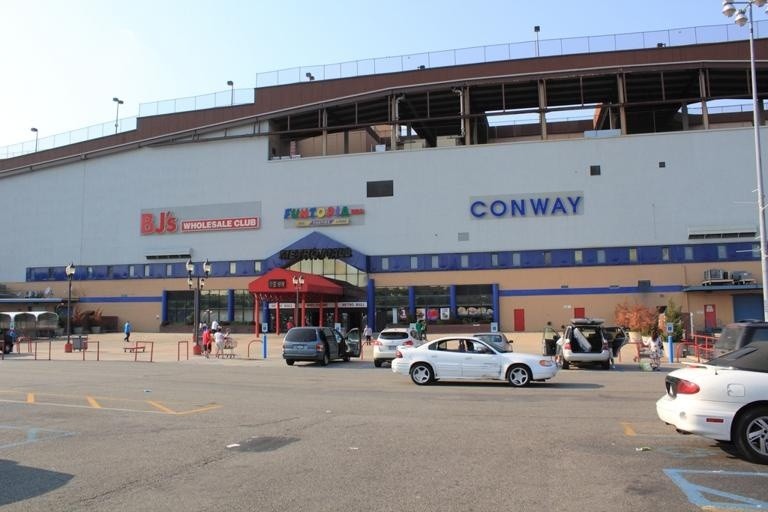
[542,317,629,370]
[373,326,422,369]
[711,320,768,365]
[0,328,13,354]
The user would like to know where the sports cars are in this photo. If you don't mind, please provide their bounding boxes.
[652,341,768,464]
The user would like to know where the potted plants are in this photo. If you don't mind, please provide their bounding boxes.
[654,296,684,360]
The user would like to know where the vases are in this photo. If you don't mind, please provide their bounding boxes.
[92,325,102,335]
[74,326,84,333]
[640,335,651,346]
[629,329,644,346]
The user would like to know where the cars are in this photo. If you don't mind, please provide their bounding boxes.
[474,333,515,355]
[391,336,557,387]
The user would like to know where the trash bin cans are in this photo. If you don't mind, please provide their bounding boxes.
[73,336,87,350]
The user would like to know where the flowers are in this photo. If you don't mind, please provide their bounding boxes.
[640,305,653,332]
[92,307,104,324]
[623,301,644,329]
[69,305,86,324]
[613,303,629,329]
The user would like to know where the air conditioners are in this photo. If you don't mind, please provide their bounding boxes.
[703,267,722,280]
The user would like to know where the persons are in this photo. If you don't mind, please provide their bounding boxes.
[285,318,294,330]
[467,342,489,353]
[415,318,424,342]
[555,337,566,367]
[123,320,132,343]
[542,321,559,362]
[559,325,567,337]
[603,328,616,370]
[363,323,373,346]
[197,318,232,360]
[643,330,665,371]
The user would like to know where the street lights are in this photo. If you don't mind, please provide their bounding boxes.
[227,79,234,106]
[532,23,541,56]
[291,275,304,325]
[111,98,125,133]
[184,257,211,355]
[29,127,40,154]
[304,71,315,82]
[187,276,204,343]
[64,262,75,353]
[719,0,767,321]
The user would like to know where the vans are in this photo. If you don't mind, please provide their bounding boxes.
[283,325,362,365]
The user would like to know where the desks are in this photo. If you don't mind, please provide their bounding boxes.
[15,336,32,355]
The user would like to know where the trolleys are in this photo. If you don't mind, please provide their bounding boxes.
[122,334,146,353]
